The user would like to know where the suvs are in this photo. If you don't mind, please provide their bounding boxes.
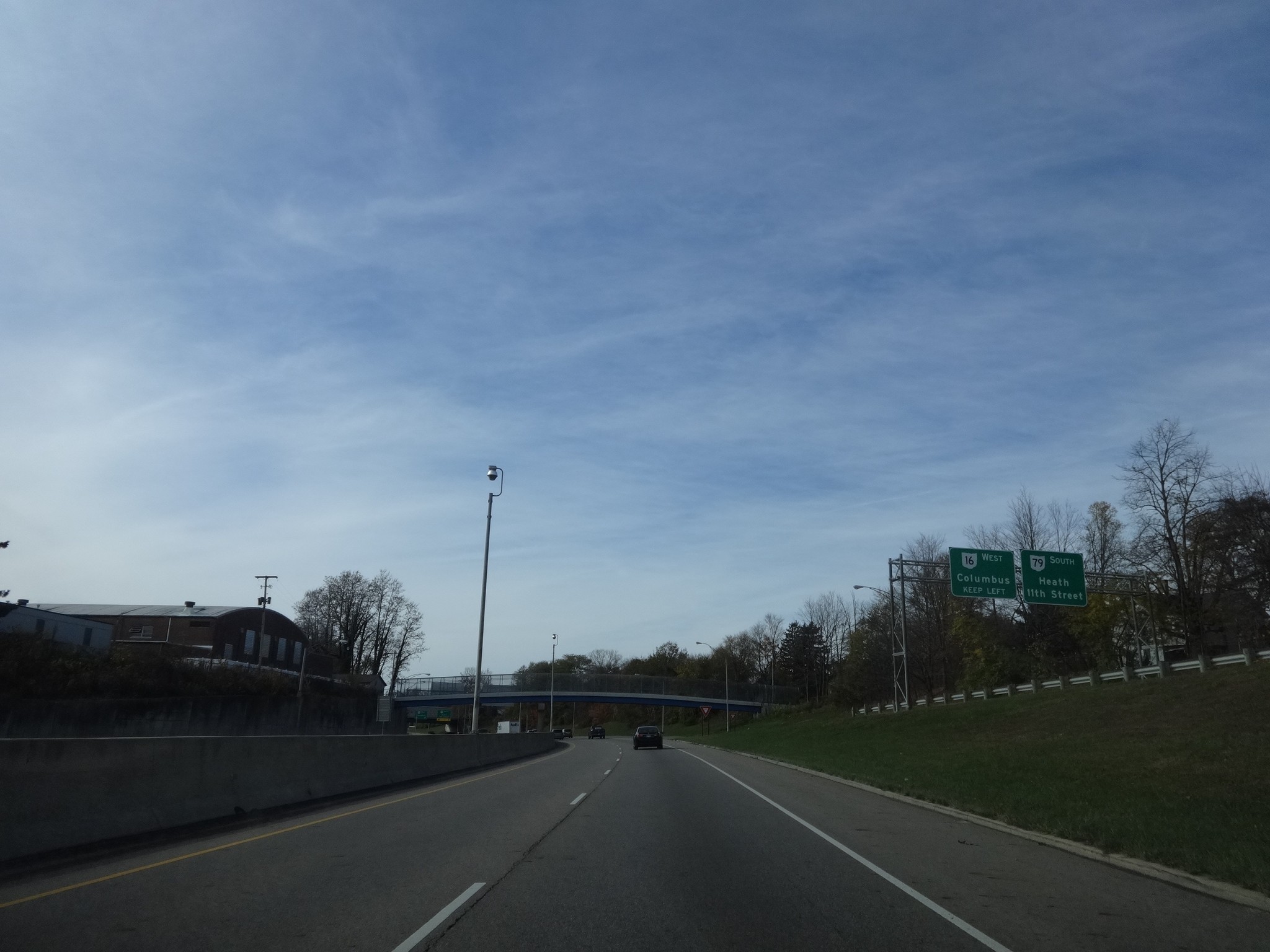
[552,729,564,740]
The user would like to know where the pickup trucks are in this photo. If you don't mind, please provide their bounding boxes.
[588,725,605,739]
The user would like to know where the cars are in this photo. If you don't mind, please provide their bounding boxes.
[476,729,490,734]
[633,726,663,750]
[527,729,538,733]
[562,729,573,738]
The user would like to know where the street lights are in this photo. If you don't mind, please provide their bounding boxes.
[696,642,729,733]
[298,639,348,696]
[853,584,909,712]
[400,673,431,697]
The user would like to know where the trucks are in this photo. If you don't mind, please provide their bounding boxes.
[495,721,520,733]
